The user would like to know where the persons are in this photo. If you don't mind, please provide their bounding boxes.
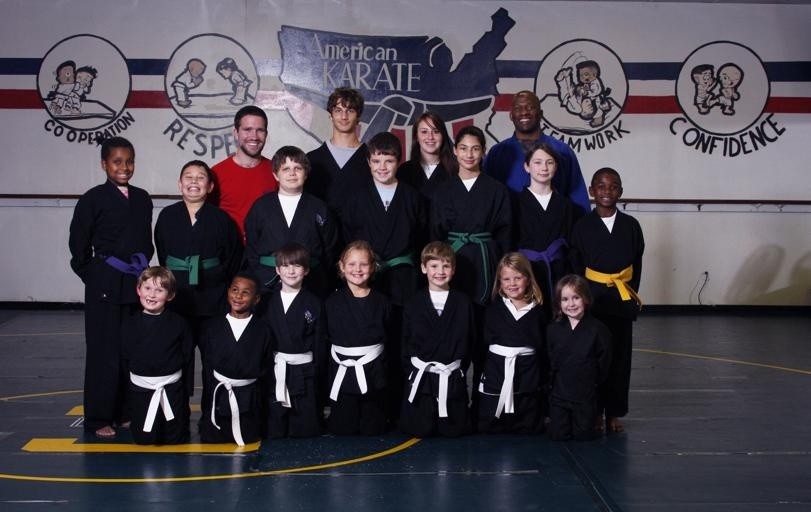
[394,110,459,210]
[544,273,614,442]
[499,142,585,304]
[68,135,154,440]
[395,240,475,440]
[194,269,277,448]
[429,128,511,307]
[472,251,545,434]
[154,160,243,430]
[566,167,645,432]
[339,131,440,306]
[481,90,591,214]
[204,106,277,247]
[265,241,321,439]
[243,145,333,307]
[319,238,394,435]
[113,265,194,445]
[303,85,372,226]
